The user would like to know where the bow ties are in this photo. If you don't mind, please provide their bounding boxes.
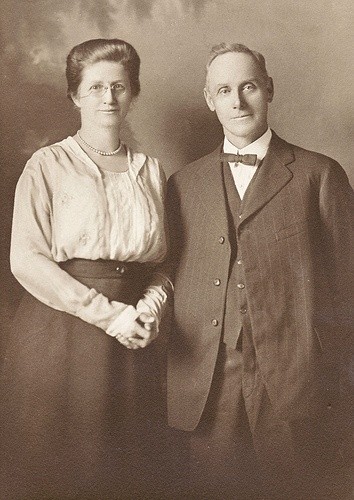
[220,152,255,165]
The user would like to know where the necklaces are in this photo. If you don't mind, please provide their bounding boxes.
[74,130,123,155]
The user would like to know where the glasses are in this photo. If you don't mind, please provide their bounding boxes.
[87,83,126,96]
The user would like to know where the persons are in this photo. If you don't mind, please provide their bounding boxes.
[166,42,354,500]
[1,38,175,500]
